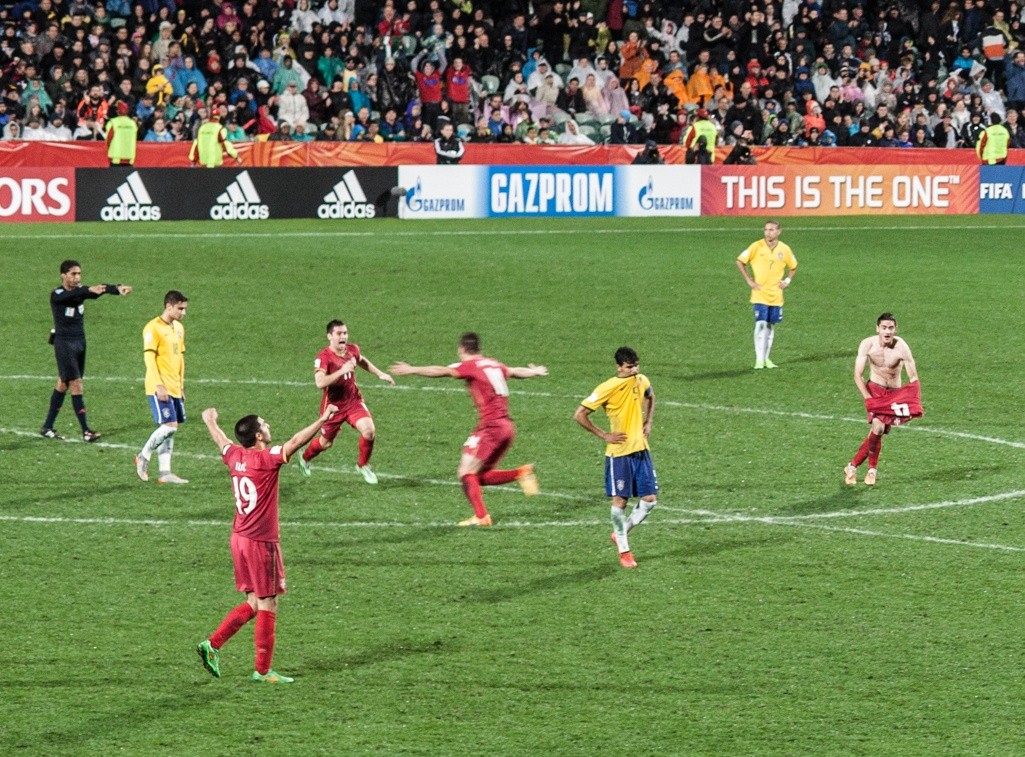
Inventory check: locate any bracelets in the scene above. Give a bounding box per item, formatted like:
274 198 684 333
784 278 791 284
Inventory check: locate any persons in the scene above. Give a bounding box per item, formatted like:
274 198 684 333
135 290 188 484
843 312 923 486
388 331 549 526
196 404 339 682
38 260 132 441
573 346 659 567
736 222 796 369
299 319 395 484
0 0 1025 166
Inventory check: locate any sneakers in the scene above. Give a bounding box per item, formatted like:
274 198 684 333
84 430 102 441
843 462 857 484
865 467 878 486
297 454 312 478
610 533 617 546
252 669 294 684
158 472 188 484
196 638 221 679
753 360 764 369
356 462 378 485
458 514 492 526
516 464 537 497
617 548 637 569
40 426 66 441
135 453 151 482
764 359 780 370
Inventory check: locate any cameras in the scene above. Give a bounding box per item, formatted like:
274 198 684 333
644 149 658 160
739 147 750 158
698 142 706 153
